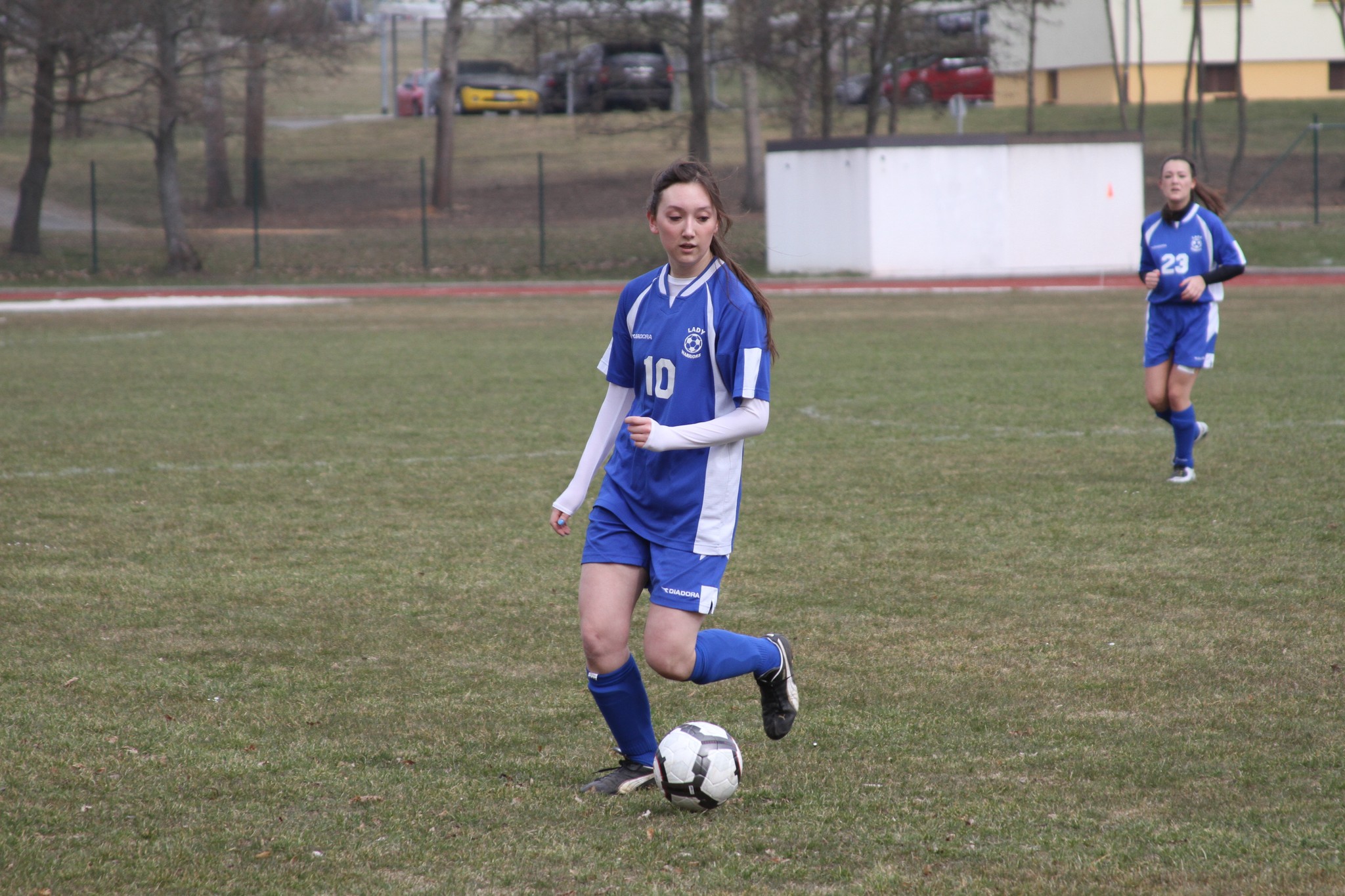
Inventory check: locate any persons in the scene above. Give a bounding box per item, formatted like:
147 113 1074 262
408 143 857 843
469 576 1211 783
1139 156 1250 482
547 155 802 793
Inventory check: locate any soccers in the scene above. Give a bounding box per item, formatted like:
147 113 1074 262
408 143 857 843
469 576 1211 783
651 721 744 813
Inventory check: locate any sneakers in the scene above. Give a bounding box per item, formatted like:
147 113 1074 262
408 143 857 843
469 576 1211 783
1167 465 1196 482
754 633 799 739
576 759 656 797
1193 421 1208 444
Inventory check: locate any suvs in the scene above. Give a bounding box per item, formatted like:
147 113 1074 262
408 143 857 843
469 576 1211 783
531 52 585 115
572 43 675 110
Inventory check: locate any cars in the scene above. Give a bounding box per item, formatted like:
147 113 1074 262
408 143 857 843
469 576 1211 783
393 66 472 131
424 58 537 116
870 45 995 100
